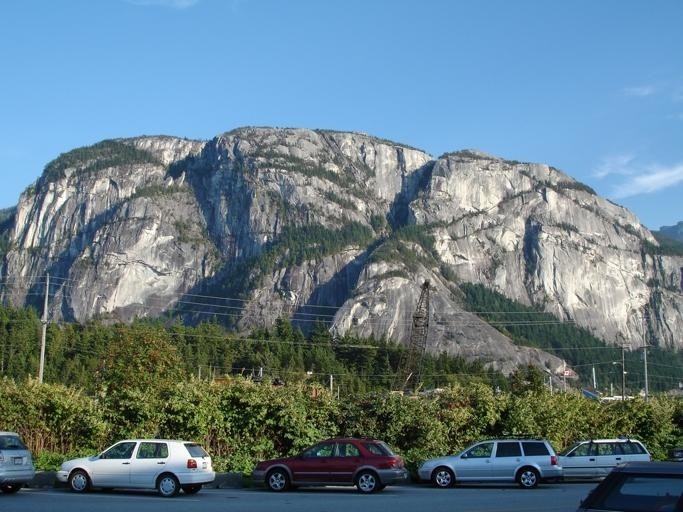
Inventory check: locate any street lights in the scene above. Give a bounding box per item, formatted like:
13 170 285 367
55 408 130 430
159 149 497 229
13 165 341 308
612 359 627 400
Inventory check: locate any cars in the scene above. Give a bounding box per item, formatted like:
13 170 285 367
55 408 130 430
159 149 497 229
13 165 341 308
256 437 408 495
578 461 683 512
418 438 564 490
54 436 216 495
559 437 651 481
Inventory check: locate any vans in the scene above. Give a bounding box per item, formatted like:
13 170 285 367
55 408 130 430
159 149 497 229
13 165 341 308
0 431 35 497
